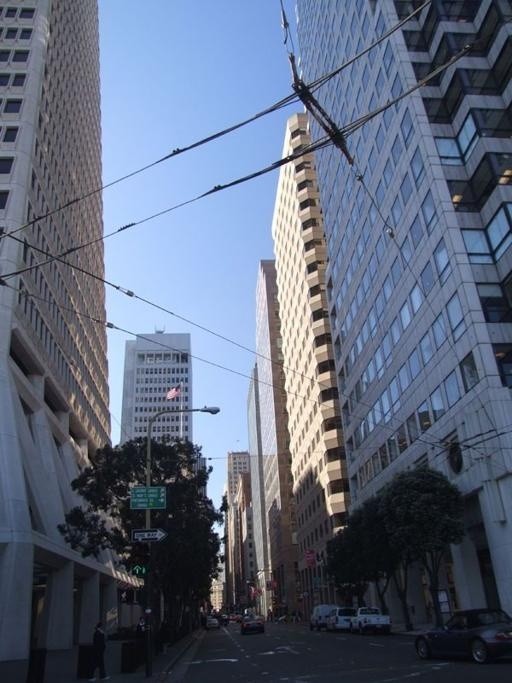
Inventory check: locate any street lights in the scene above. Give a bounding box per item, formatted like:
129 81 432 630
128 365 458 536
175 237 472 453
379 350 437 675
143 405 226 678
164 380 192 439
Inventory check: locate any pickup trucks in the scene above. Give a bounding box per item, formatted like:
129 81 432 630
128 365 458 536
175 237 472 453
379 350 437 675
349 607 393 635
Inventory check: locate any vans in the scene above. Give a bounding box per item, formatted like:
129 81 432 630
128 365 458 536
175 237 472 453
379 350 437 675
309 604 357 632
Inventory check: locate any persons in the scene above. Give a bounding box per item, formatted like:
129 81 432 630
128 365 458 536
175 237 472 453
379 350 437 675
86 622 112 682
136 616 145 631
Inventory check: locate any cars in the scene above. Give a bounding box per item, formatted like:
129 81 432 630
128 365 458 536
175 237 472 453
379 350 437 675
206 613 265 634
415 608 512 664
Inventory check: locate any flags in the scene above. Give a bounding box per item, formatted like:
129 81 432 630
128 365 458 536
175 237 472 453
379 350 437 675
166 382 182 402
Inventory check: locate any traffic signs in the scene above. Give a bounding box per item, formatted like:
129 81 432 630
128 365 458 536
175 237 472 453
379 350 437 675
131 528 169 544
130 486 166 510
